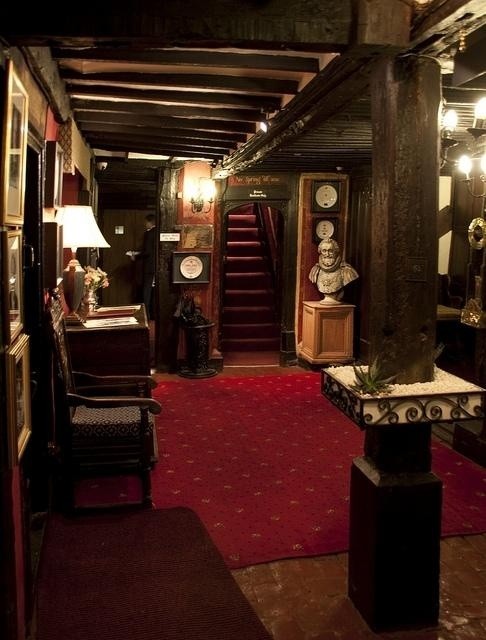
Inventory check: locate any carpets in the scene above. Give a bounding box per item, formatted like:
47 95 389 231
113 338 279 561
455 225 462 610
70 359 485 572
25 497 278 639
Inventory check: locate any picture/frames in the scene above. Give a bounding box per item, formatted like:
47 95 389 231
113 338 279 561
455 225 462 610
9 327 36 465
1 58 30 230
46 136 65 211
172 251 213 287
310 215 339 247
310 175 342 215
78 187 95 209
3 225 26 351
43 218 65 291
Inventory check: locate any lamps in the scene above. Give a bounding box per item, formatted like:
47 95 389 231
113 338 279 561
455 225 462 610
185 176 217 216
455 150 485 204
259 118 273 134
467 96 486 142
55 202 111 326
441 107 455 170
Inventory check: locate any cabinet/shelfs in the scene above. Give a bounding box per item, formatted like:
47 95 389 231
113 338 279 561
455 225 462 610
297 298 357 370
179 321 218 379
65 299 155 401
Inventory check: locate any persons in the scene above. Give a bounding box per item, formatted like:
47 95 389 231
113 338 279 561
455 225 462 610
125 213 158 319
309 235 360 305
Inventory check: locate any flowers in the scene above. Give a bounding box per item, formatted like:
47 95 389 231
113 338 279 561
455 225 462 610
81 259 109 290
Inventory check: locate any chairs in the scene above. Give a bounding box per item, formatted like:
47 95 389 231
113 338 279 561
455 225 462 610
46 301 162 512
436 272 465 358
